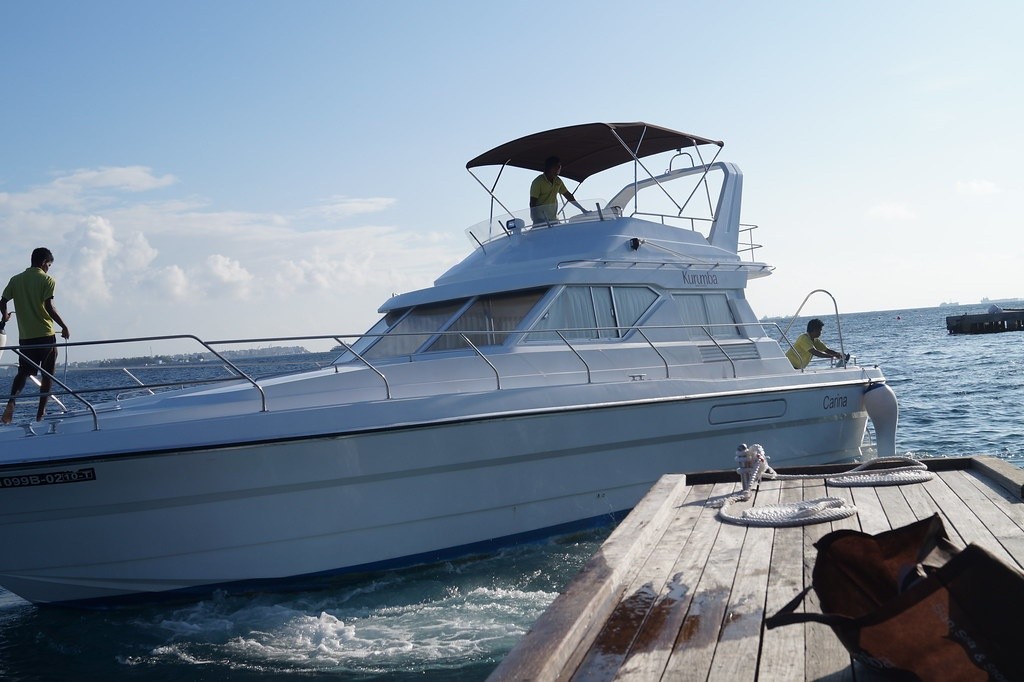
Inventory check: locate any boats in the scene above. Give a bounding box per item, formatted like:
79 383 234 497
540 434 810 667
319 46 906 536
0 122 899 617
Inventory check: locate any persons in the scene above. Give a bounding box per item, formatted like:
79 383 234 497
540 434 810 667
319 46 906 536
0 247 69 424
529 157 593 228
785 319 847 370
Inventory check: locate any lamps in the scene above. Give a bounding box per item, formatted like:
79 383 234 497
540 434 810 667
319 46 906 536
630 237 640 250
506 218 525 234
